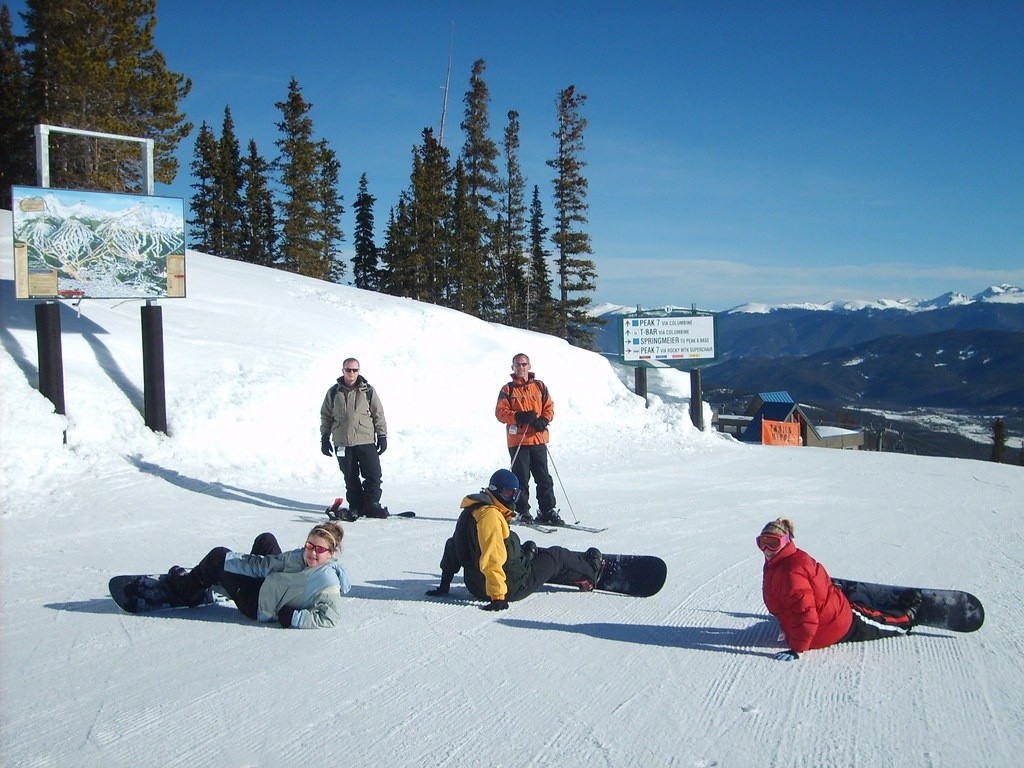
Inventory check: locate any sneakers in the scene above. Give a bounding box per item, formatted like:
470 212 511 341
366 504 389 518
517 510 533 522
349 503 364 519
575 547 602 583
521 540 536 564
538 509 561 523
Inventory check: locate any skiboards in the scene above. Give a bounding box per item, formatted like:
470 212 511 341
510 520 610 534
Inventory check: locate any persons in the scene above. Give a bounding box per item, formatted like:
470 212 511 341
169 522 351 628
757 518 921 661
495 353 562 523
425 469 602 612
320 358 389 519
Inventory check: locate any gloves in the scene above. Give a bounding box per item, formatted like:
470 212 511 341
772 650 799 661
321 439 333 457
376 435 387 455
533 416 549 432
483 599 509 611
515 410 536 424
206 550 226 573
278 605 295 627
425 589 443 597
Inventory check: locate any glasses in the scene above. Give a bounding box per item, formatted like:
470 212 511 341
514 362 528 367
756 533 781 551
500 487 521 503
344 367 359 373
305 541 331 553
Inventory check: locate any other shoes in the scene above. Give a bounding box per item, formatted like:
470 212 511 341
168 566 192 606
902 590 922 628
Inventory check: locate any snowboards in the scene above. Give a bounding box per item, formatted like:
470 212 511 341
829 576 985 634
521 544 668 598
387 511 416 517
107 572 232 614
